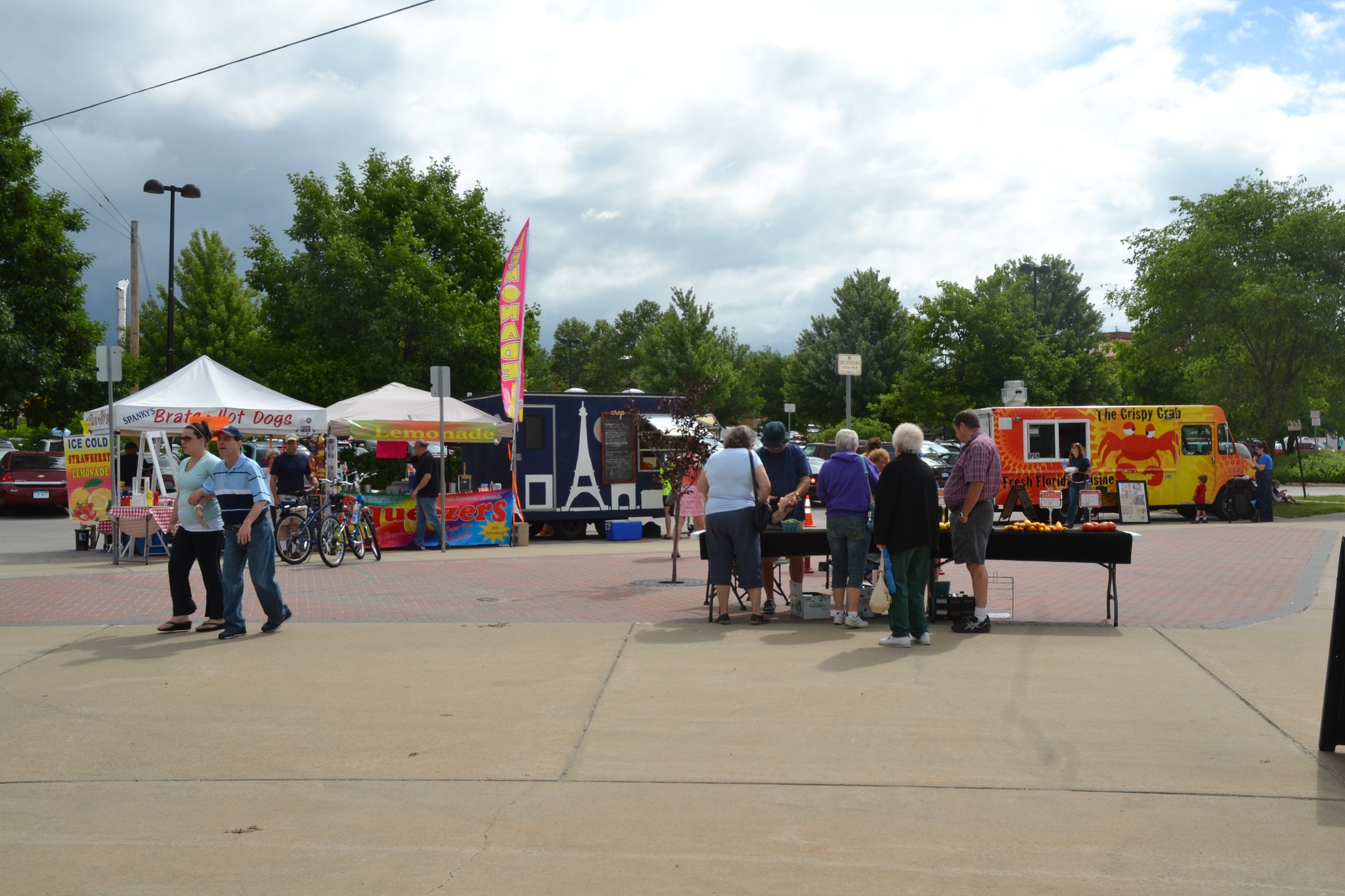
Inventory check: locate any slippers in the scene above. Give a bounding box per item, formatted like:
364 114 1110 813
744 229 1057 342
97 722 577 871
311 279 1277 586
196 621 226 631
662 534 671 539
671 553 681 558
158 621 192 631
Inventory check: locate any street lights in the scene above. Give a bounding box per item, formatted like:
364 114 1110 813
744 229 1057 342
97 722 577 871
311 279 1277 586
560 335 580 390
144 175 203 456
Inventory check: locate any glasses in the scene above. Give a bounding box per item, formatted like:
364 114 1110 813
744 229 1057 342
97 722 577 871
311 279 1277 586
413 446 420 449
1256 448 1261 452
180 436 199 442
877 460 887 465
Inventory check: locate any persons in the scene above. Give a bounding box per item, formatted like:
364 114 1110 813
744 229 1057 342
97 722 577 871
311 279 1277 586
860 437 890 463
1271 478 1303 505
1191 475 1209 523
1249 440 1274 522
659 441 711 559
1063 442 1094 529
193 425 294 641
754 421 813 615
817 428 870 629
874 423 940 648
943 409 1001 635
270 433 320 561
263 446 288 556
407 439 451 550
114 442 153 490
696 424 772 625
639 450 661 482
156 418 226 633
865 447 890 581
918 449 939 613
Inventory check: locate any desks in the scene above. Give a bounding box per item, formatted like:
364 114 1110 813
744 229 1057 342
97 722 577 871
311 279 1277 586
107 505 180 567
699 525 1132 627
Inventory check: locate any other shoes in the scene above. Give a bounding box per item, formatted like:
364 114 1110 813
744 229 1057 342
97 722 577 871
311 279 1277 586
749 614 771 625
1295 501 1302 504
1064 523 1073 529
715 613 730 624
290 552 302 559
281 550 289 561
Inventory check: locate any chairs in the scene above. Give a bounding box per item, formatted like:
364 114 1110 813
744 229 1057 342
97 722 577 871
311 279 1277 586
703 556 790 611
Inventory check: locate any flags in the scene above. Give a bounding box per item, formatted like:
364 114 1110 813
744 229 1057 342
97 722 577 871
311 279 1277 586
500 220 530 423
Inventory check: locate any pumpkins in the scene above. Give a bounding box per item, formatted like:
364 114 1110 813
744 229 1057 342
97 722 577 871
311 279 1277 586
1004 520 1067 532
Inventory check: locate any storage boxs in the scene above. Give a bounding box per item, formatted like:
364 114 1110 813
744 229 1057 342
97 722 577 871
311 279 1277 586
781 520 800 532
605 519 642 541
858 585 882 618
789 580 831 620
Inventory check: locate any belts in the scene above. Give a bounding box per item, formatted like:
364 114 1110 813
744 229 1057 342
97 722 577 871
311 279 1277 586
949 498 991 513
222 513 267 533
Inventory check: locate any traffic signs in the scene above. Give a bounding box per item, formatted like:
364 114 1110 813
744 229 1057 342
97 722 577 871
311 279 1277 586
1287 418 1302 431
1311 418 1321 426
1310 410 1320 418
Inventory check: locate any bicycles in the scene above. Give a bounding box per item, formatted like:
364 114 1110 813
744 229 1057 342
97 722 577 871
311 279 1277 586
317 469 382 569
272 469 358 565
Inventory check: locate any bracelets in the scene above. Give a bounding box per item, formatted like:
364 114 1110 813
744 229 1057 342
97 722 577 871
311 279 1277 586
309 475 317 484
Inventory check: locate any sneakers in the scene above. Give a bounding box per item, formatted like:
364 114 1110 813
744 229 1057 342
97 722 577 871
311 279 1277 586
953 614 990 626
911 631 931 645
1191 520 1199 523
218 625 247 639
951 615 991 633
878 634 911 648
844 613 869 628
402 542 423 551
762 599 776 613
260 604 293 633
833 609 847 624
1202 519 1208 523
431 544 450 550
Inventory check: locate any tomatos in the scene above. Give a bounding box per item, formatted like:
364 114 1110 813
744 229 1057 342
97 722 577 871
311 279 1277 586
1082 521 1116 532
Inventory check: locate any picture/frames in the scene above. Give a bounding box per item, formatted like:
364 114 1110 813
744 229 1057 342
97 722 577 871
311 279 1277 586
1115 480 1151 524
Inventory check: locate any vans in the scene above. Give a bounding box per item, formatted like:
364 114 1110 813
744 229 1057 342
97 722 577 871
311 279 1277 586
1180 440 1253 468
962 403 1259 522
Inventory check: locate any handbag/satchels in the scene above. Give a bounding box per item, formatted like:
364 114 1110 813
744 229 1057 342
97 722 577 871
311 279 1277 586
751 500 770 532
868 547 892 613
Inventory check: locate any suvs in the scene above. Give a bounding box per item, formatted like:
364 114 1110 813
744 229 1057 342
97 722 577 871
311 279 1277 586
233 442 314 483
33 426 72 452
1293 441 1345 454
126 452 183 494
406 441 451 462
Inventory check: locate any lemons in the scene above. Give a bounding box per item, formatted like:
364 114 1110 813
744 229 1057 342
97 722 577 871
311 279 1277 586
70 478 111 510
404 504 442 532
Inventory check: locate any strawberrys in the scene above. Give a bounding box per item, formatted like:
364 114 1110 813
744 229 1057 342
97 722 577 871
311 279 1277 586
70 502 97 521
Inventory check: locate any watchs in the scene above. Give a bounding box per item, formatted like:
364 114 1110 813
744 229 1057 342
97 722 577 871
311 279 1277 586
792 491 801 499
959 512 967 519
193 504 203 511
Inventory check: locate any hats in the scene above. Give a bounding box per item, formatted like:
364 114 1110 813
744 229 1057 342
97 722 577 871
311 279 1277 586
284 433 299 443
125 442 139 452
759 421 790 448
264 453 279 464
213 425 244 442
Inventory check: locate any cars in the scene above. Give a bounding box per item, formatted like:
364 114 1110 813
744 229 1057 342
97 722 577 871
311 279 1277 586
0 438 19 463
0 450 69 512
699 419 961 510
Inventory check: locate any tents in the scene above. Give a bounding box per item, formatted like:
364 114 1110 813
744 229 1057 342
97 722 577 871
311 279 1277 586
324 381 516 547
83 354 328 561
